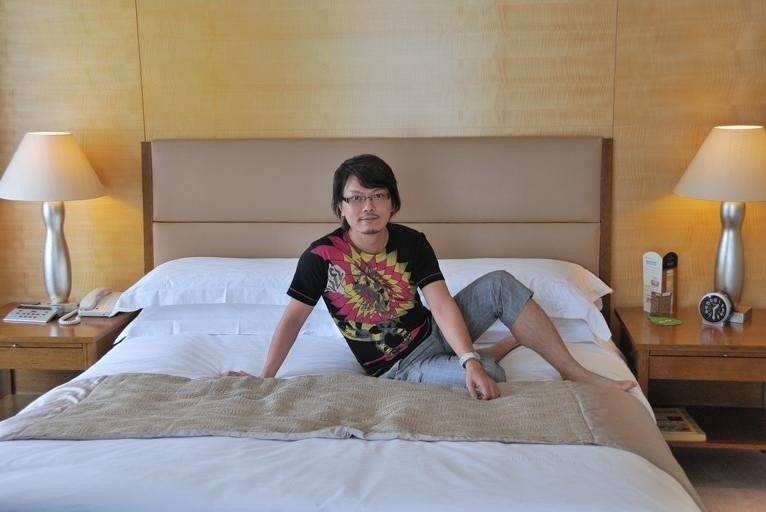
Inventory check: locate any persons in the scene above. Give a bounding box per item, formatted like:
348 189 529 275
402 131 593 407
217 154 637 399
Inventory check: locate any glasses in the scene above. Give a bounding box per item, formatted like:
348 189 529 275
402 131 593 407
337 190 390 205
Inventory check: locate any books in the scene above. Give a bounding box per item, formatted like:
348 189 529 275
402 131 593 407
653 406 705 443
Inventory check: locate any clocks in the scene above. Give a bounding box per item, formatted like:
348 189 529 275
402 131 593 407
699 293 732 328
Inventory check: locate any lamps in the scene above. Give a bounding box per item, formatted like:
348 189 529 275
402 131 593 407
1 133 109 314
673 127 766 325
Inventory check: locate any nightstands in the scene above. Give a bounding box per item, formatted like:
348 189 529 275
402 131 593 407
0 303 141 397
617 307 765 452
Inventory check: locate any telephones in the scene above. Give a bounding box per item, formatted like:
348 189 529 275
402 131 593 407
59 286 124 325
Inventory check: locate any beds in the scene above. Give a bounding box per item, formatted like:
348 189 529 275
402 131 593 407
0 134 709 510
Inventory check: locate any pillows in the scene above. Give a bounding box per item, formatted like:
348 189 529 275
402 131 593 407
110 257 330 314
418 258 614 343
475 316 595 349
111 305 346 345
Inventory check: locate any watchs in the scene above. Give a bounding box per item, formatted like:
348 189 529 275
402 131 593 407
457 350 481 368
